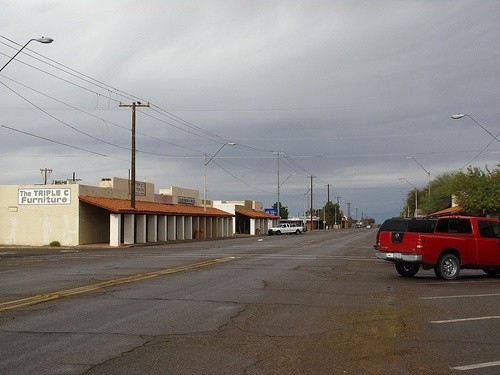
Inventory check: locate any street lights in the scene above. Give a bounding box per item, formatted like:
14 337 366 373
304 185 316 226
276 171 296 218
400 177 418 218
203 142 237 212
405 156 431 202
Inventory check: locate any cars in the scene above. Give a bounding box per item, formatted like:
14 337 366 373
352 224 361 228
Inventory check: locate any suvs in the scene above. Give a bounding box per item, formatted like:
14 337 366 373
373 217 440 252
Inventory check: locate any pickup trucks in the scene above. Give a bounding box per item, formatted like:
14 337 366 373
377 215 500 281
267 224 304 236
